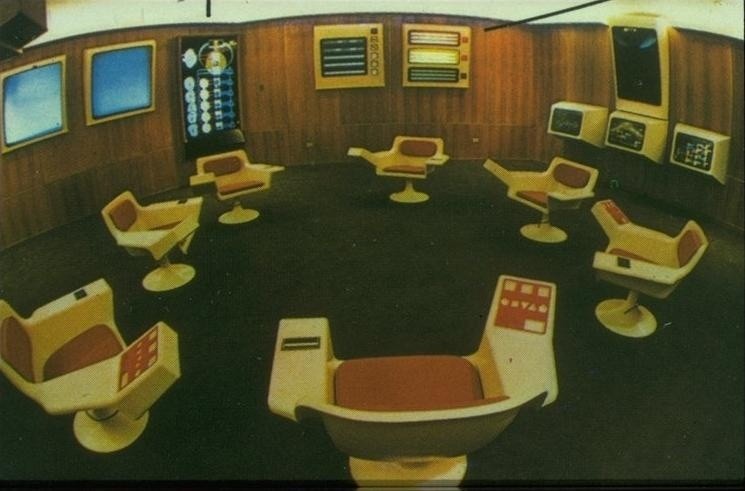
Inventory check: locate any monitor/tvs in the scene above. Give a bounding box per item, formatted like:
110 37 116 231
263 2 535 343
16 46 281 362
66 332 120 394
606 14 671 121
82 39 158 127
0 54 70 155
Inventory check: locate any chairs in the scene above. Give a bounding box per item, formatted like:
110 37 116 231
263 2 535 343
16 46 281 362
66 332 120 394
264 274 563 486
588 199 708 340
482 156 600 244
2 279 184 455
341 135 450 205
191 149 285 224
100 193 203 295
